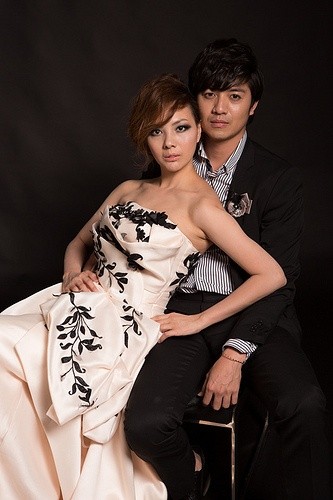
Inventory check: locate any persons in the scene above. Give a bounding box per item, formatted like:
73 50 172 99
124 37 332 499
1 75 287 500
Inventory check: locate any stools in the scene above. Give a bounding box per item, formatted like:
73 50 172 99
184 379 269 499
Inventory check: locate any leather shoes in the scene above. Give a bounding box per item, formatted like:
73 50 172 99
179 452 212 500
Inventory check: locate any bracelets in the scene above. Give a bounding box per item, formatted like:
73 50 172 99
221 351 243 365
